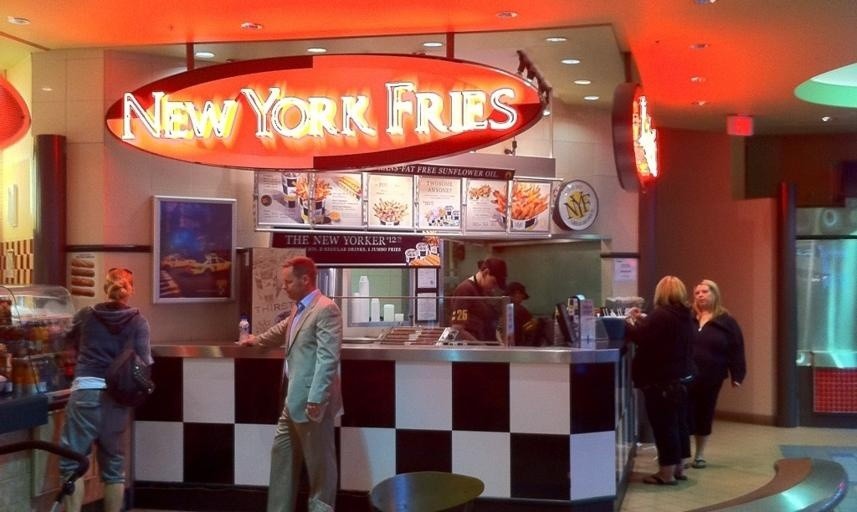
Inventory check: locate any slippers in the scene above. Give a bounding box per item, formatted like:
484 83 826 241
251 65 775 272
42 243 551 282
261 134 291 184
644 457 706 484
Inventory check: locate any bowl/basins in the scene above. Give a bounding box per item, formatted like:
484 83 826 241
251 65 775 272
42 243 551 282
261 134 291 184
497 212 538 231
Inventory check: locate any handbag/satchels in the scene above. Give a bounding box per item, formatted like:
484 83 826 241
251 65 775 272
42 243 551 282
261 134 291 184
106 349 154 406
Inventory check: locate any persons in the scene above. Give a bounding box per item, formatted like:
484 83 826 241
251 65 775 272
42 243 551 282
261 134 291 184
503 281 539 346
627 276 702 486
682 279 747 470
57 265 155 512
448 259 508 348
242 256 349 512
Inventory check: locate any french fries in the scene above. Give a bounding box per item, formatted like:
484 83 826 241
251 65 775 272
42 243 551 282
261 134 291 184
512 182 548 219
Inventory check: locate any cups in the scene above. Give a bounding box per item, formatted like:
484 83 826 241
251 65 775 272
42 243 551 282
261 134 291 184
379 218 401 226
351 275 403 322
427 204 460 227
405 242 439 265
281 173 333 224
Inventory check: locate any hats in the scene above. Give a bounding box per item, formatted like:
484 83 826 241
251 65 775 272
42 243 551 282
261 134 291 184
486 258 508 289
508 281 531 300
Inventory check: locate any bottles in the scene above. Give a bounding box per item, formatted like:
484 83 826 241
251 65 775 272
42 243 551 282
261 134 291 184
0 315 76 395
238 313 249 339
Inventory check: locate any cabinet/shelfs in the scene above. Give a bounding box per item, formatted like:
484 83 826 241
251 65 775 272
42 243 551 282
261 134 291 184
0 282 82 398
